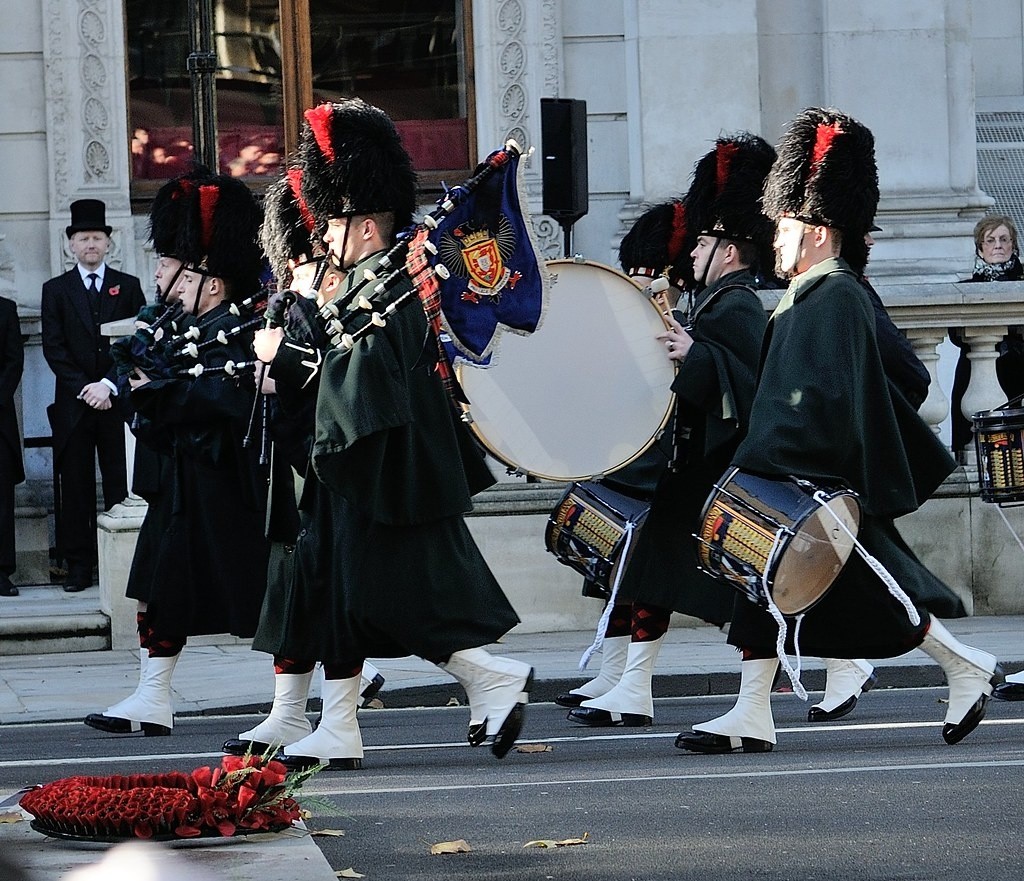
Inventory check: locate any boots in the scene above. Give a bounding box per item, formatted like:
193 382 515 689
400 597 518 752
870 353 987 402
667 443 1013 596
992 670 1024 701
83 647 183 736
262 671 363 769
315 659 385 726
222 666 316 755
555 635 633 707
436 662 492 747
444 646 534 759
808 657 877 720
566 632 666 727
917 612 1003 745
673 657 781 752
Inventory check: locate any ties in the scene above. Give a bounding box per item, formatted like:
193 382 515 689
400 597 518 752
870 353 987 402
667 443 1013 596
87 274 100 310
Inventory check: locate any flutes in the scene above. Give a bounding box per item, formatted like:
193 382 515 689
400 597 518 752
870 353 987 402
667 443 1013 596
150 135 522 468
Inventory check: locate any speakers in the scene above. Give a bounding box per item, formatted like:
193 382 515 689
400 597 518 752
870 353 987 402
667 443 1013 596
540 98 589 229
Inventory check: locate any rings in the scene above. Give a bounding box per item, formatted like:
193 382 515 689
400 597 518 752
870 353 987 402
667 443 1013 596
669 342 673 349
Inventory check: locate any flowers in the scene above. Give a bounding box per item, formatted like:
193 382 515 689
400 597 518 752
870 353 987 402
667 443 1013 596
20 728 357 845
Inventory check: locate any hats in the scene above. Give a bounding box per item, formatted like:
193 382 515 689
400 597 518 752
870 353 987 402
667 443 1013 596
66 199 113 239
620 101 882 292
142 95 417 287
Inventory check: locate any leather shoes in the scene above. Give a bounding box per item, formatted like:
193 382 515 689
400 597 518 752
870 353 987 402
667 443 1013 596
0 578 18 596
64 568 92 591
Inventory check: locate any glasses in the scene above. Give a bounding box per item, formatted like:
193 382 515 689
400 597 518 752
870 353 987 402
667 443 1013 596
981 235 1012 246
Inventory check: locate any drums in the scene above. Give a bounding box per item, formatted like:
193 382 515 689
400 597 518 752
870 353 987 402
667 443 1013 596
689 464 865 620
453 255 680 482
970 408 1024 504
545 481 653 595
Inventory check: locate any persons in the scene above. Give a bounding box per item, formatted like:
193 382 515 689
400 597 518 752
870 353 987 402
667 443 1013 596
0 296 26 596
992 669 1024 702
222 173 488 753
272 98 535 762
567 132 877 727
675 108 1005 755
555 203 881 720
948 214 1024 452
39 199 146 589
84 163 386 752
